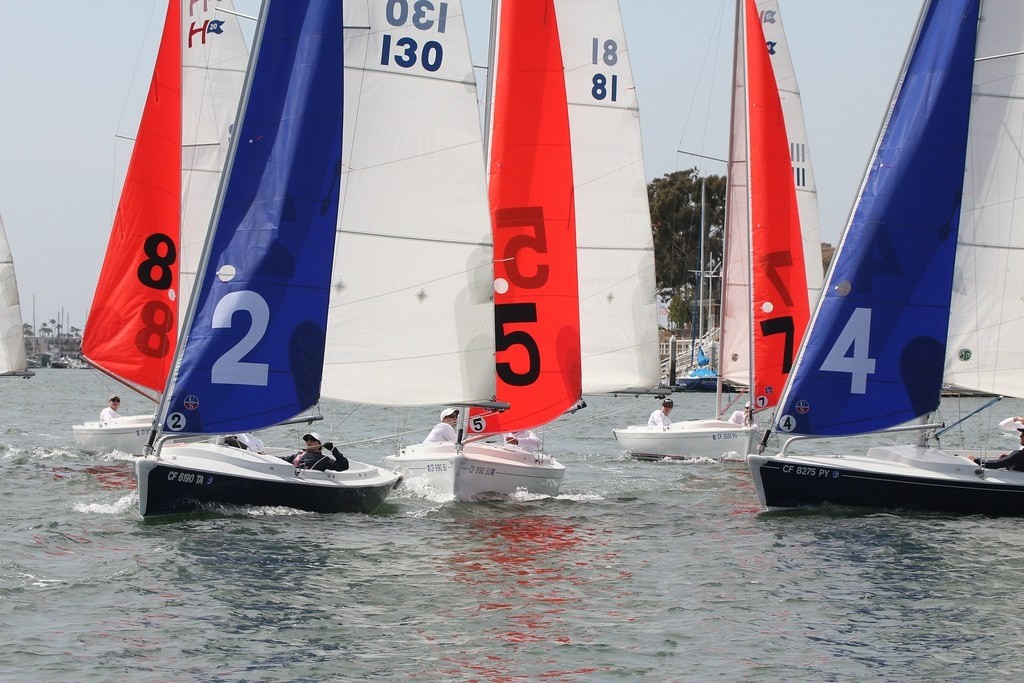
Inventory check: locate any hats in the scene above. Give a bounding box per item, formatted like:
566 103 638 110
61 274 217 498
108 394 120 402
440 408 460 422
302 432 322 444
745 400 754 410
661 398 674 407
1017 427 1024 433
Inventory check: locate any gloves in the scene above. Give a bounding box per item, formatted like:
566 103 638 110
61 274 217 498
323 442 335 452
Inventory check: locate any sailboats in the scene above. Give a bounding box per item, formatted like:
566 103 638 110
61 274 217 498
0 214 95 377
78 0 663 519
613 0 1024 517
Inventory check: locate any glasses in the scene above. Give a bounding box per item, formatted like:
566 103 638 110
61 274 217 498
447 414 458 418
305 437 318 442
745 407 749 409
663 404 672 408
110 398 120 403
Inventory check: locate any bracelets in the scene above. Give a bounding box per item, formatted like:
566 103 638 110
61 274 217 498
516 439 518 445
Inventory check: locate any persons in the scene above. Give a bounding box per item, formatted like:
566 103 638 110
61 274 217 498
99 393 121 421
423 408 459 444
503 430 540 451
275 432 349 471
729 401 755 423
647 399 674 429
968 416 1024 471
